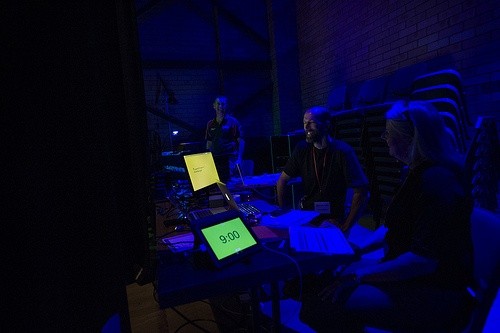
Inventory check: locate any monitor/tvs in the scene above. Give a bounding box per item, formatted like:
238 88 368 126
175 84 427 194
201 216 258 267
183 151 221 197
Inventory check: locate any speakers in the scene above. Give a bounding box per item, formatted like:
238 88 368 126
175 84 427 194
269 130 305 209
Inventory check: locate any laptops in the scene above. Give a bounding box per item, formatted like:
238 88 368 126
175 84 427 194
218 181 261 217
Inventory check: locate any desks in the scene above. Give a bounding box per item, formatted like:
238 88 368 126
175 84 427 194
156 172 357 333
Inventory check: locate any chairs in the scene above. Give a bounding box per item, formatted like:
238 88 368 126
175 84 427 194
269 132 301 210
463 207 499 333
331 69 494 227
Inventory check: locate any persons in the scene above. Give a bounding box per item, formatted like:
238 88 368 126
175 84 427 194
204 96 240 160
302 102 469 333
276 110 370 232
